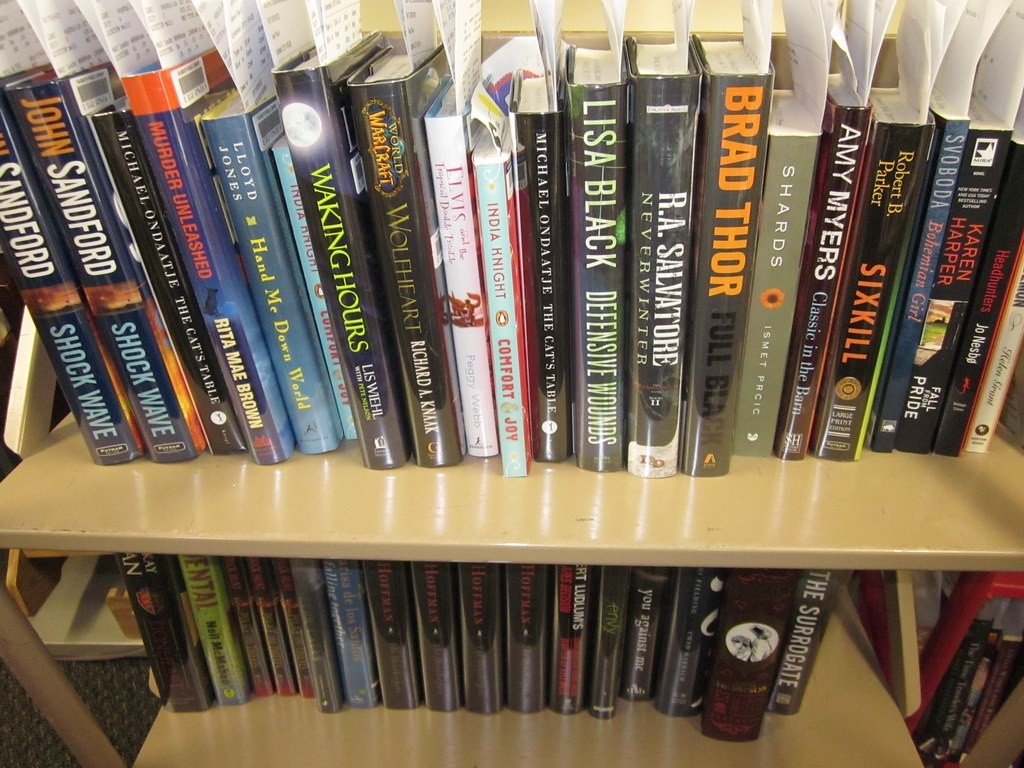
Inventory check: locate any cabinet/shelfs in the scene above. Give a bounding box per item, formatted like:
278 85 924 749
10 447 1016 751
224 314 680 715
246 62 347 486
0 302 1024 768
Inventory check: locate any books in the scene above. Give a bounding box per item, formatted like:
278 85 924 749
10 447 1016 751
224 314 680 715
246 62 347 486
116 553 839 743
0 35 1023 479
848 569 1023 768
